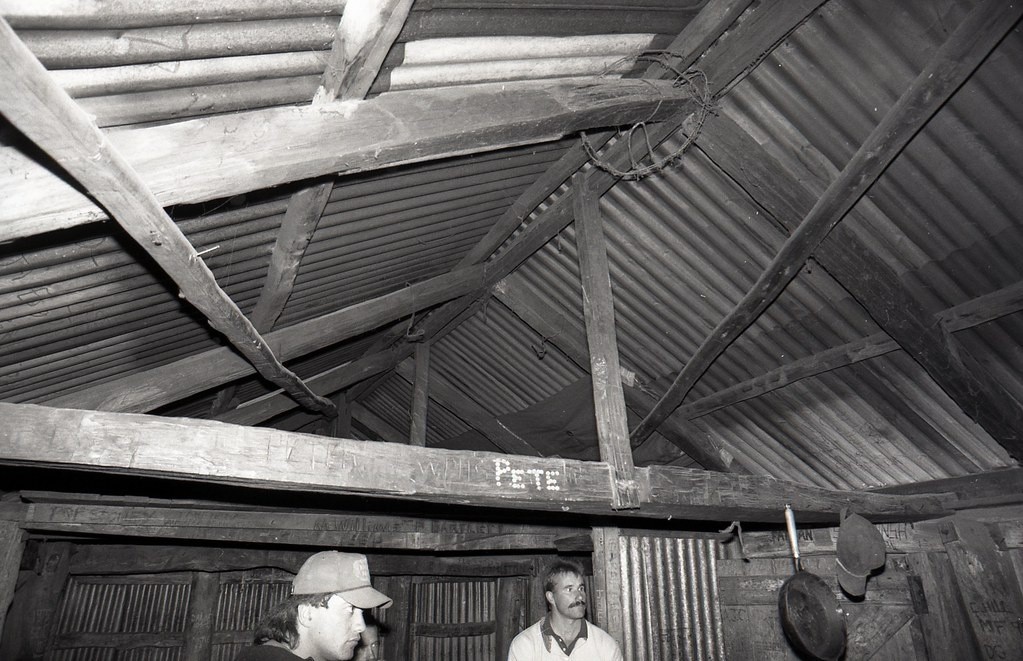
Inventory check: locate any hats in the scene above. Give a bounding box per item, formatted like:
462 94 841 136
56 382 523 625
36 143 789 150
837 506 886 596
291 550 393 609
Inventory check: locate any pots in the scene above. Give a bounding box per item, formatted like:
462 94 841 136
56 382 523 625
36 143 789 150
778 504 848 661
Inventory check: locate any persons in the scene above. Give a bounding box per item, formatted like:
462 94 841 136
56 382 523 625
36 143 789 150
231 550 393 661
507 560 625 661
